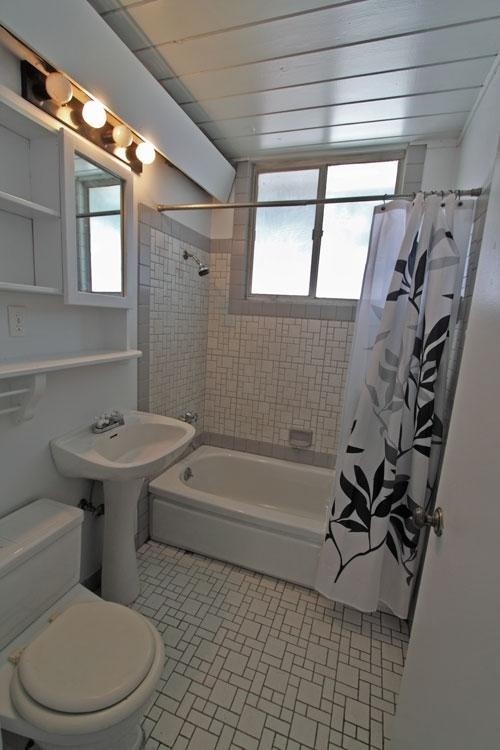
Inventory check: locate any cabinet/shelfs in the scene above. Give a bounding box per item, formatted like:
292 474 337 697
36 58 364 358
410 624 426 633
0 185 60 298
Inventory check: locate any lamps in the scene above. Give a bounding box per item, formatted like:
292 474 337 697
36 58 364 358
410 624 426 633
21 58 159 175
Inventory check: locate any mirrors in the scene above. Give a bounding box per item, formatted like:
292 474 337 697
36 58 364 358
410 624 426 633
58 124 133 309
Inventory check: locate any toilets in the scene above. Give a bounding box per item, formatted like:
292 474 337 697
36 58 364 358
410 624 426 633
0 498 165 749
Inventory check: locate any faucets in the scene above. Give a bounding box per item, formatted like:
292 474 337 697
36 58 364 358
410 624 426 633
179 408 196 422
93 407 122 428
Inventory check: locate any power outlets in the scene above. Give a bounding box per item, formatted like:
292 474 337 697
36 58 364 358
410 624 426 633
8 304 28 338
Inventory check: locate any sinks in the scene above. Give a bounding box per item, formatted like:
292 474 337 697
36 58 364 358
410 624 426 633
49 410 195 482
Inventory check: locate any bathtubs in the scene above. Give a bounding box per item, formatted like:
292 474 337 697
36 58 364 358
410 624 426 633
148 444 336 590
